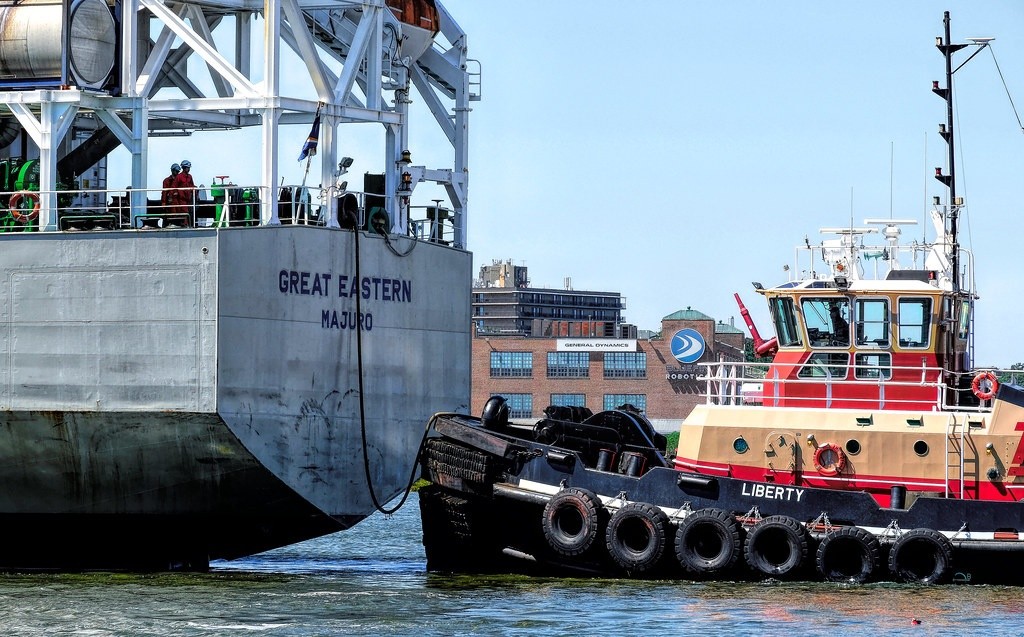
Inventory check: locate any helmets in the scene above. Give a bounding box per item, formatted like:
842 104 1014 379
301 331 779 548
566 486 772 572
181 160 191 167
171 163 181 172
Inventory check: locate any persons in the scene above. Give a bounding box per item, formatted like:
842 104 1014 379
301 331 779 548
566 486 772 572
829 306 848 346
161 160 196 229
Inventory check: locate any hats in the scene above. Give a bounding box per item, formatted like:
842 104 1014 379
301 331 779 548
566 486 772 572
829 307 839 313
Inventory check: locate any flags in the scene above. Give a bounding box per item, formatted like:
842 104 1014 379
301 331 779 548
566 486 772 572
298 107 320 161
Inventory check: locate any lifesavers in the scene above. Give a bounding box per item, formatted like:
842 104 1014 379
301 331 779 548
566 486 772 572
10 193 40 222
816 527 879 582
813 444 846 477
542 487 601 556
744 516 807 575
606 504 667 572
674 508 739 574
889 529 952 586
972 373 998 399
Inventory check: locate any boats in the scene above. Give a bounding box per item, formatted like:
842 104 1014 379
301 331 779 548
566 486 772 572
419 9 1024 587
0 0 481 554
